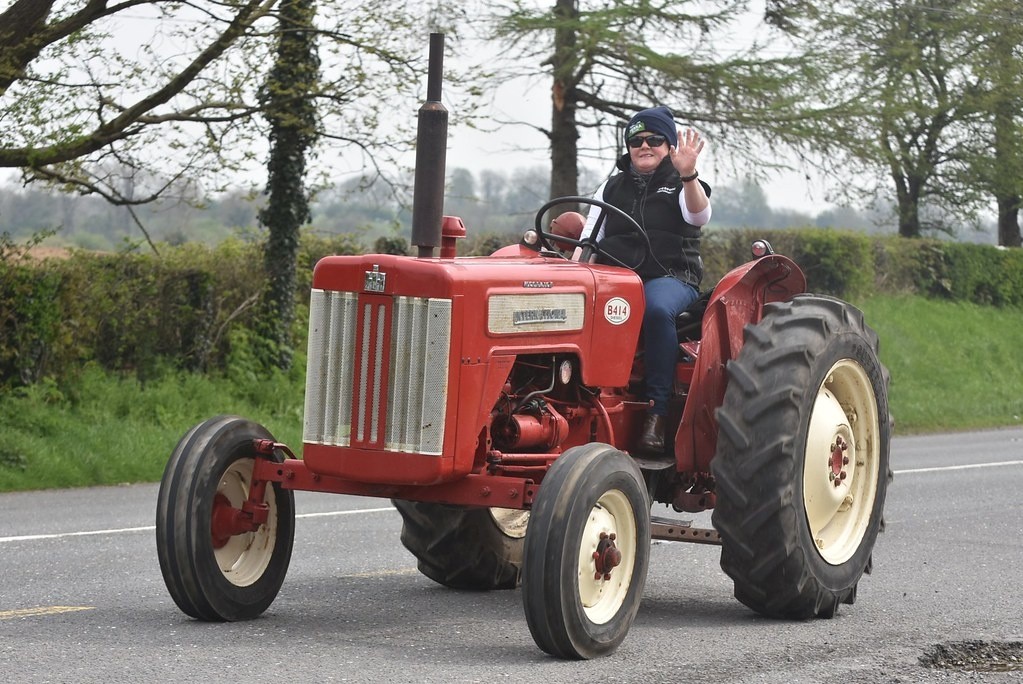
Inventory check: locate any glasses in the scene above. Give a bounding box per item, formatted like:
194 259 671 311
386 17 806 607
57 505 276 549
628 135 667 148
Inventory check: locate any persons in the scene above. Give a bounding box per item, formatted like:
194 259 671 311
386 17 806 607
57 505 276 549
516 106 712 457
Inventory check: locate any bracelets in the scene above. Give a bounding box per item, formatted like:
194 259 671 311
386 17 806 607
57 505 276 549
680 169 698 182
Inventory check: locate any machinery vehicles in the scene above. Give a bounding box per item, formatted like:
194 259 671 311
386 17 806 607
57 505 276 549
153 30 896 662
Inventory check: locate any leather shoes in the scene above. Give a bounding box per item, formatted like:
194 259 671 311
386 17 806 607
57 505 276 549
635 411 666 454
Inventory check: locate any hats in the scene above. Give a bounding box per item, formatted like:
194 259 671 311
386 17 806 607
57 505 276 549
624 106 677 152
